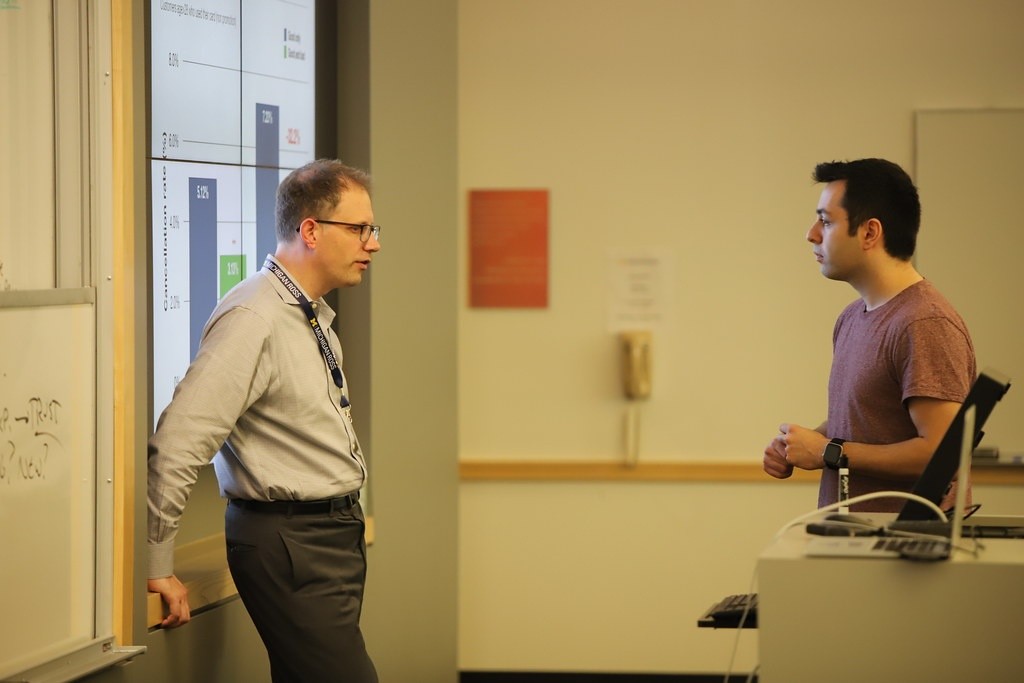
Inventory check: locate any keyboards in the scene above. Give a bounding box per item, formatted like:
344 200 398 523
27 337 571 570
710 593 758 620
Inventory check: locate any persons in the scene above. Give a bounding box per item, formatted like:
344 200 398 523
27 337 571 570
764 158 976 519
147 159 382 683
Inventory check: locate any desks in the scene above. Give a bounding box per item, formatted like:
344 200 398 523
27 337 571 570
753 501 1024 683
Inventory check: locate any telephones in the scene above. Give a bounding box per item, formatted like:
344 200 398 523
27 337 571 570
616 330 653 400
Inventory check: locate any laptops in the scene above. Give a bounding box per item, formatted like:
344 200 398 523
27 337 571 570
802 402 975 559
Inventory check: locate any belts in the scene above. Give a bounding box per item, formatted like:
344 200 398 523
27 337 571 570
231 492 359 513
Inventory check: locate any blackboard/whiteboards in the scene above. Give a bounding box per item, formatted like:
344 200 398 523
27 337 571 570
0 288 115 683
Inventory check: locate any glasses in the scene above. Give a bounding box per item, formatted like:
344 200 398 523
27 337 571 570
296 220 380 242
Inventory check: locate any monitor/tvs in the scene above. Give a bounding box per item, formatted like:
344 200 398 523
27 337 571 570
896 367 1011 523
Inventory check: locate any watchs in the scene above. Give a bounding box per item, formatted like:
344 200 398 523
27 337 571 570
824 438 846 470
